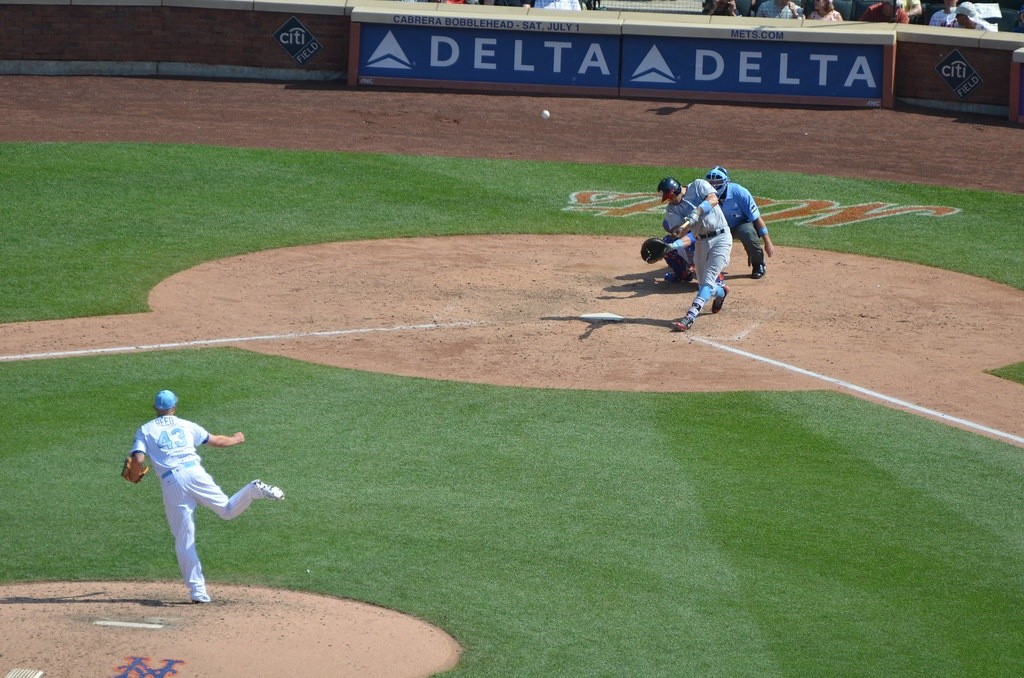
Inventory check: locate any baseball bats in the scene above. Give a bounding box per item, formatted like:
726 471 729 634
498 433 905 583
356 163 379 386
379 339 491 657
673 221 689 237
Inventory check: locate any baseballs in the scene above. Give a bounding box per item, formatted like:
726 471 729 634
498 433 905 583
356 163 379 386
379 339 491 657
541 110 550 119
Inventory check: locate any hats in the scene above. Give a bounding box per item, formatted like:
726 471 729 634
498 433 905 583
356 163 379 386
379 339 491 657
950 1 977 16
155 389 180 410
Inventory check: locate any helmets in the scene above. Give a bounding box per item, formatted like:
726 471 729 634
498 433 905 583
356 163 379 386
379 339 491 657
704 165 729 196
657 176 681 204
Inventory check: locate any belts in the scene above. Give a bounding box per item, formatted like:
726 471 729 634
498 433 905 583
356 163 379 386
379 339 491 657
161 460 199 479
695 229 725 240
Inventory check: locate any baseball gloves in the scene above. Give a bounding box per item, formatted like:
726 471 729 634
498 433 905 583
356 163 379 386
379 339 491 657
640 237 670 264
121 457 150 483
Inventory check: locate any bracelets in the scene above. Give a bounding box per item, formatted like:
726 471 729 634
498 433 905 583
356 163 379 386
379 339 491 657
759 227 768 235
699 200 712 214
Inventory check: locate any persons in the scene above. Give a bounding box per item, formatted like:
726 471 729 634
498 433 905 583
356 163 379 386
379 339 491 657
703 0 1024 36
640 232 725 285
657 177 734 331
704 166 775 280
120 390 285 605
420 0 596 12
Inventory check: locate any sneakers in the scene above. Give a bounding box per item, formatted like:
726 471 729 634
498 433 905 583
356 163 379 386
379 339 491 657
664 272 686 282
712 286 729 314
715 278 725 287
672 315 693 331
254 479 285 500
191 594 210 603
751 263 766 278
